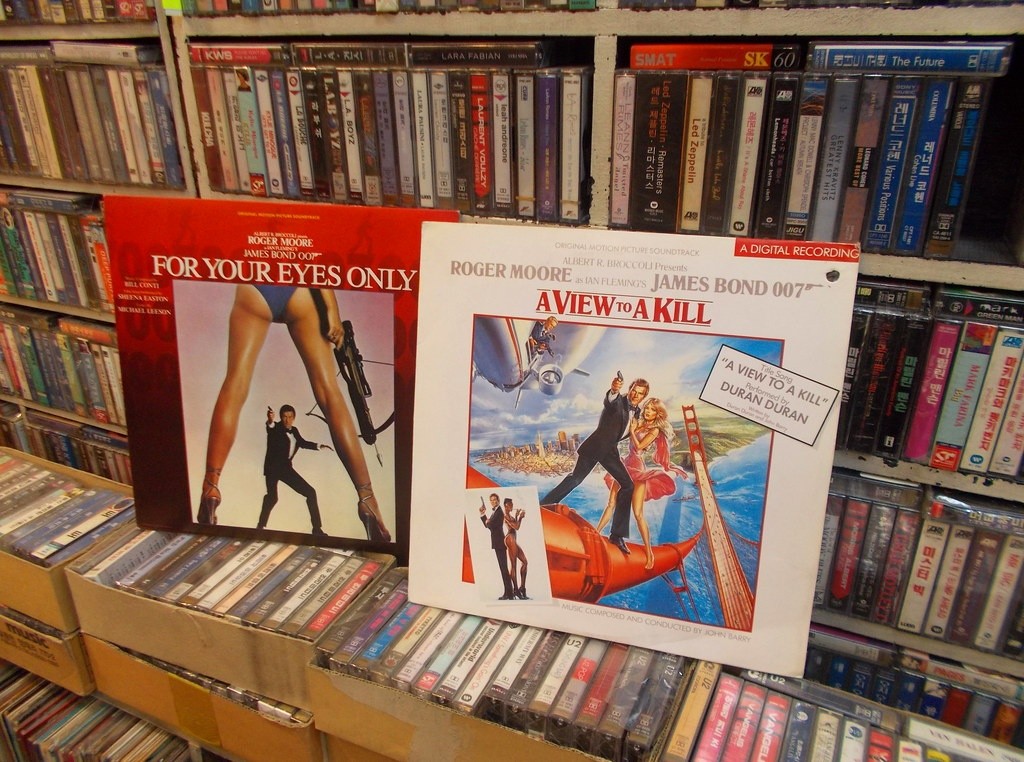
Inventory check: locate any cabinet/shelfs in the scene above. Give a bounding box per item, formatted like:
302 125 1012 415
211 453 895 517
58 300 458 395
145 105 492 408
0 0 1024 762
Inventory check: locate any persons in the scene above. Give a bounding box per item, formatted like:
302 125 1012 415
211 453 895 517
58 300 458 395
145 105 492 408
257 404 334 536
196 283 391 542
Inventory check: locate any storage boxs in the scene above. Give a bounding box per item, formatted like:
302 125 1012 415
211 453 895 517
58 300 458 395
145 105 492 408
0 444 607 762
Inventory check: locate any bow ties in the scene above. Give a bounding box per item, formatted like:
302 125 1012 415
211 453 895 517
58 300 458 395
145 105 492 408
492 507 495 511
628 405 638 412
285 427 293 434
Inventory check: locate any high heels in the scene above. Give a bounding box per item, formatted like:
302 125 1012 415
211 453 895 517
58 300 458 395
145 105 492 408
514 587 531 599
197 468 222 526
356 484 389 542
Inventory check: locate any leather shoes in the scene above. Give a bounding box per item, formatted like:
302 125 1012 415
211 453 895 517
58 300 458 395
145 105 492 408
499 593 514 600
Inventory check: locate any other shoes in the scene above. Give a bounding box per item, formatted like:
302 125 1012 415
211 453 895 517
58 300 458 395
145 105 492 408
312 529 328 536
